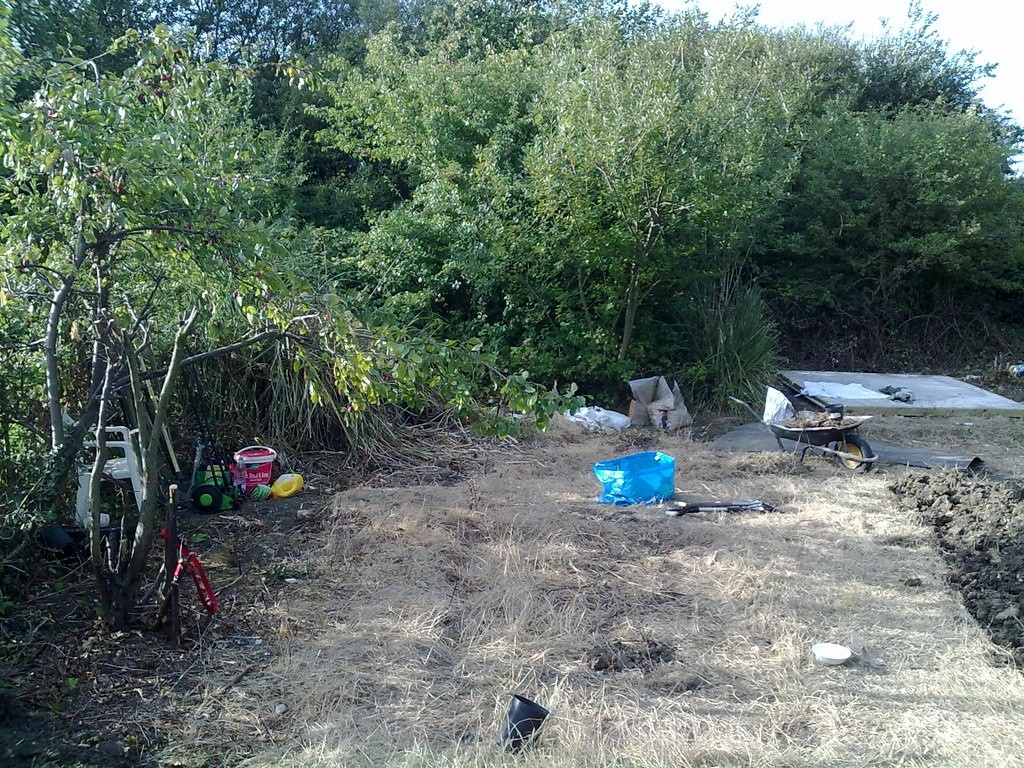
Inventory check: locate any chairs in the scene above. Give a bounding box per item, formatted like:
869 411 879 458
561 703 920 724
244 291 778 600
76 426 142 524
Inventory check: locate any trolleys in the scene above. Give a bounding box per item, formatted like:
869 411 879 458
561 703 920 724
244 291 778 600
729 386 878 474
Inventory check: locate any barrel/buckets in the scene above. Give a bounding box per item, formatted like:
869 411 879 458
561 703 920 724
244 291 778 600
825 403 844 424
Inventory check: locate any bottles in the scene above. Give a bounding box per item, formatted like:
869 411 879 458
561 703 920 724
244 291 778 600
234 457 247 495
